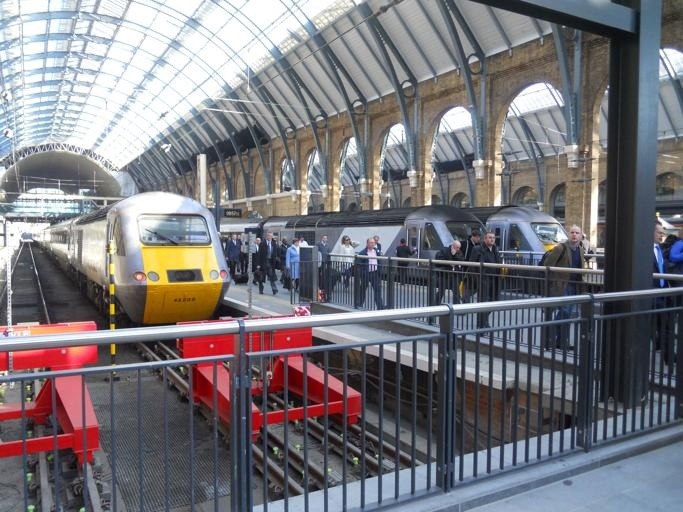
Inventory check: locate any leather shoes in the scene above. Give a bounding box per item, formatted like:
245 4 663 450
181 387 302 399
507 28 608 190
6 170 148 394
260 290 278 295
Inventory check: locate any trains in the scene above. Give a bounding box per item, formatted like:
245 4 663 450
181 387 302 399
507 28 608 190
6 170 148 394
41 192 230 326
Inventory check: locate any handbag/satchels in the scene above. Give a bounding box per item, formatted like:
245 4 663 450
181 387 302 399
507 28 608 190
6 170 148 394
283 276 294 288
538 244 566 265
253 269 263 282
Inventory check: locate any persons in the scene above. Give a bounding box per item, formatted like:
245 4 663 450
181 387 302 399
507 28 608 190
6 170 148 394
542 225 592 351
220 230 388 310
395 238 418 286
652 222 683 365
436 230 503 337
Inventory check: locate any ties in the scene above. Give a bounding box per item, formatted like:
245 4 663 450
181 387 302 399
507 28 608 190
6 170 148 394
655 245 665 287
268 242 270 258
